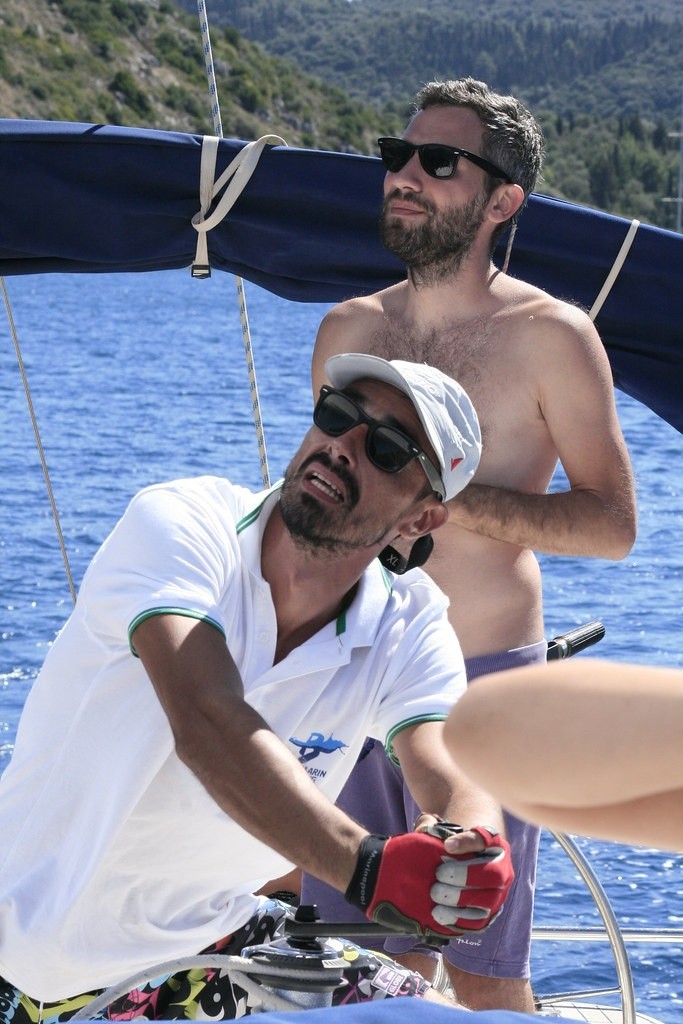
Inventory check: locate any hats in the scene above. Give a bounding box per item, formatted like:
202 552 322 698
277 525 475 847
324 352 482 502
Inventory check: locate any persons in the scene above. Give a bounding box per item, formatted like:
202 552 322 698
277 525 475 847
300 75 640 1014
441 658 683 854
0 353 515 1024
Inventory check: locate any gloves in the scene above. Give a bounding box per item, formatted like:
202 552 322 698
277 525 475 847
342 814 514 941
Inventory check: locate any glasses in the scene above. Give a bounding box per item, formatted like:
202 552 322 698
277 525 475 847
375 132 511 191
312 382 446 505
